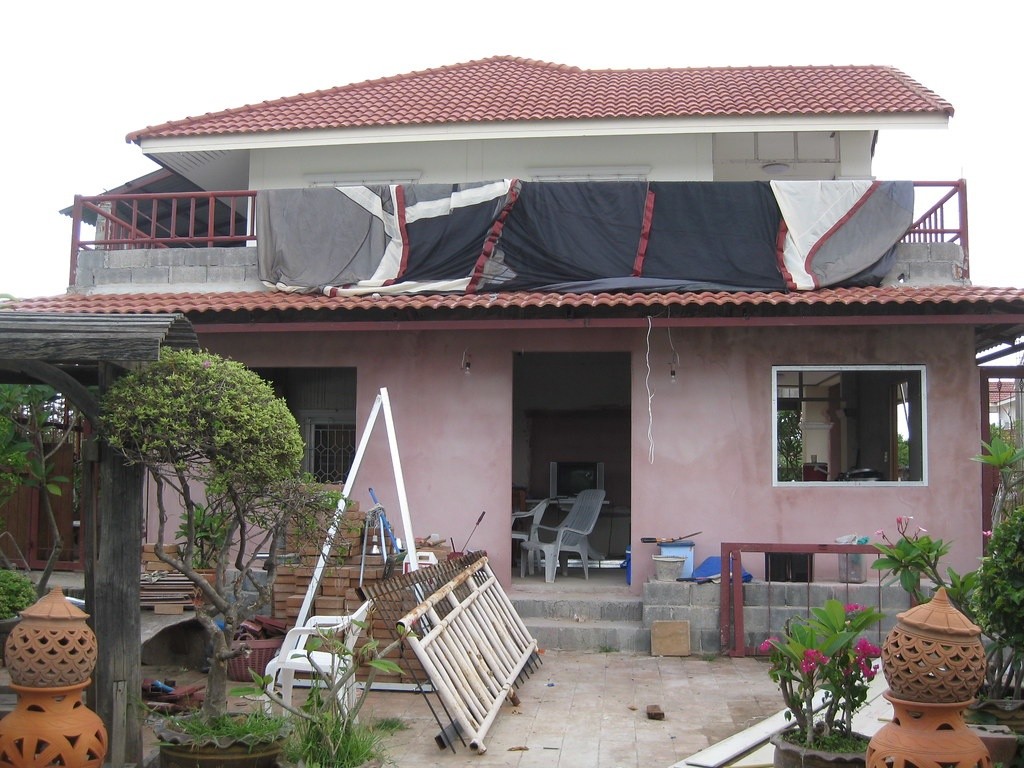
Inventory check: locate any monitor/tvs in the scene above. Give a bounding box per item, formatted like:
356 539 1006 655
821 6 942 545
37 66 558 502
550 461 605 500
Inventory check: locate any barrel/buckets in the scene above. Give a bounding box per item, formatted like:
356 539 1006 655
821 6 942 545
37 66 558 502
620 545 631 585
651 554 687 582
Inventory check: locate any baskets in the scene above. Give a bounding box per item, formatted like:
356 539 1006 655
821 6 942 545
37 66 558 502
226 633 282 682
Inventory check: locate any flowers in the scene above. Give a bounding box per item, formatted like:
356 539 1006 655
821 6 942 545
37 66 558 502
761 597 880 745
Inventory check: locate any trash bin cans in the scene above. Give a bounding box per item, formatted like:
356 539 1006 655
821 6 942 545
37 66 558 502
839 544 867 584
657 540 695 579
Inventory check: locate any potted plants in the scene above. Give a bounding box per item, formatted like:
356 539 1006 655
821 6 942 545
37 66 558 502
0 570 39 667
98 345 365 767
227 600 419 768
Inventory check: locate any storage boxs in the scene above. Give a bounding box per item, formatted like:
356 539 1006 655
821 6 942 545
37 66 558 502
838 553 867 583
766 552 815 582
802 462 828 481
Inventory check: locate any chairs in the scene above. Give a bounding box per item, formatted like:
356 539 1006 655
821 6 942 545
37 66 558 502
263 600 373 724
520 489 606 584
510 497 549 575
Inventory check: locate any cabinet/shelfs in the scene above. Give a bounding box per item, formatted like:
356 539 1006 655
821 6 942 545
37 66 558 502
523 502 630 559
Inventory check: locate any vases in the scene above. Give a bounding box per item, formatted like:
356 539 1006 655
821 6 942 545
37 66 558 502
769 726 872 767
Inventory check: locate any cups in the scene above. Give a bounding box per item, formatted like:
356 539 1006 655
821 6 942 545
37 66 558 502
811 454 817 464
